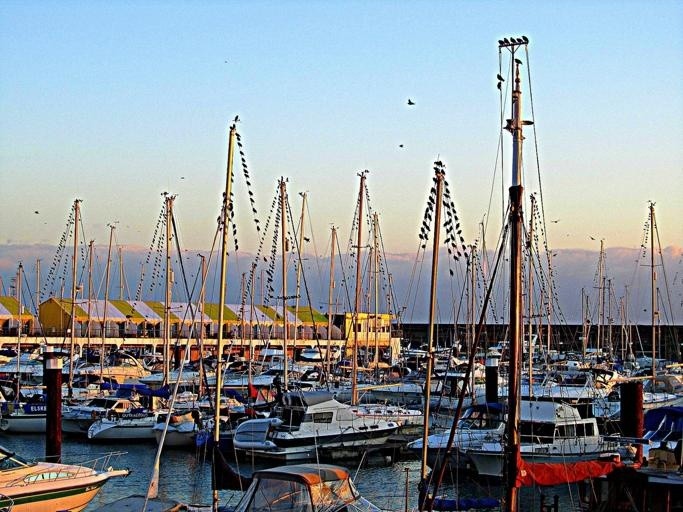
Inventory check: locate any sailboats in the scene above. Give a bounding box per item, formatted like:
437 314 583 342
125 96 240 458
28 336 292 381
0 33 682 511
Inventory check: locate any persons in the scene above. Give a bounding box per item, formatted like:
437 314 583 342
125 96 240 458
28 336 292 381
448 348 468 398
272 372 282 395
392 364 402 379
